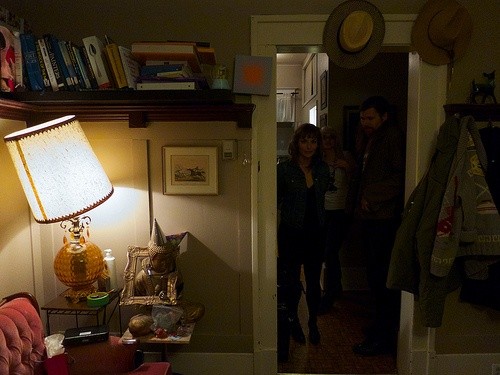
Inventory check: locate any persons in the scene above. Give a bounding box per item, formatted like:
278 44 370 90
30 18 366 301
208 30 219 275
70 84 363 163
278 123 330 344
353 97 404 356
320 125 354 314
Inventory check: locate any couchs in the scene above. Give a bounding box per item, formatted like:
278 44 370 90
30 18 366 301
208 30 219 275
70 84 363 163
0 291 171 375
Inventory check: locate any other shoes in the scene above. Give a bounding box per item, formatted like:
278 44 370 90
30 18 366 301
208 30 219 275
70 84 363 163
308 325 321 344
292 325 306 344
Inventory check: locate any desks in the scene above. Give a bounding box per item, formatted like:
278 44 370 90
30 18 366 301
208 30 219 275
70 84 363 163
40 289 123 339
117 323 197 375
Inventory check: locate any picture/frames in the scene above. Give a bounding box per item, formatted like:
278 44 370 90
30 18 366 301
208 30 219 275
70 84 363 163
120 246 182 305
320 70 327 110
160 144 221 197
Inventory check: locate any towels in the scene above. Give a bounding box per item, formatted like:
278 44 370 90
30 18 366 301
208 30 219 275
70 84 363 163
275 89 294 125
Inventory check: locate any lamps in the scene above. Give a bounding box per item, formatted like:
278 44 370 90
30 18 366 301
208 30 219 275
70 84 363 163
3 114 115 302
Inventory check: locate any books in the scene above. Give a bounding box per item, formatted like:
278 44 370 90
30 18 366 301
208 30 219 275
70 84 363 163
0 22 218 93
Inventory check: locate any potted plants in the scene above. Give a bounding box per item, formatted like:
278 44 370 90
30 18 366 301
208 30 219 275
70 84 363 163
207 61 229 89
